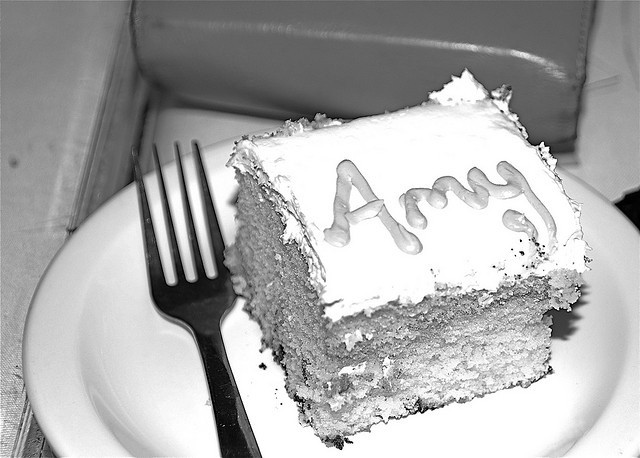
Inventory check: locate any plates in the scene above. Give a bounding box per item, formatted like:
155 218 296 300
23 130 638 458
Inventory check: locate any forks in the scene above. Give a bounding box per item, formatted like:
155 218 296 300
130 137 264 458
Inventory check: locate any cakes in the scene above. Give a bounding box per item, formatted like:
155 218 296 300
222 65 594 451
